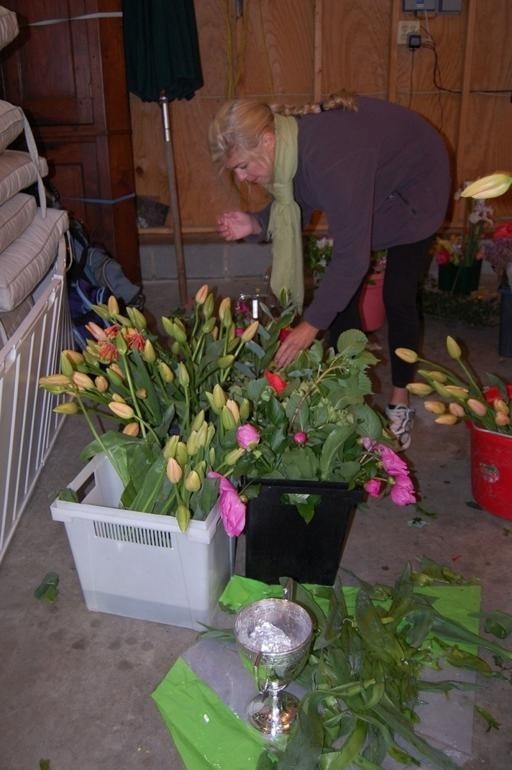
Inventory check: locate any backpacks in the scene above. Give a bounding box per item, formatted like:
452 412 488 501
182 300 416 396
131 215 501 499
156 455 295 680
61 215 146 355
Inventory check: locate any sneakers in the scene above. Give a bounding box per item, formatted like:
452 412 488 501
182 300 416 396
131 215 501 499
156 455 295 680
384 403 417 454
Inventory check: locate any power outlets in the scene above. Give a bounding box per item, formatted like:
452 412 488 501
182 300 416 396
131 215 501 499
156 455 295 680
395 20 420 46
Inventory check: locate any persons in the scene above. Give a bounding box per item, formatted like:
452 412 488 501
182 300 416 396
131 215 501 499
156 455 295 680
208 90 457 454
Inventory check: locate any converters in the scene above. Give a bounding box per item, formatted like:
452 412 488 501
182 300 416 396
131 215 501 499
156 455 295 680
407 32 421 48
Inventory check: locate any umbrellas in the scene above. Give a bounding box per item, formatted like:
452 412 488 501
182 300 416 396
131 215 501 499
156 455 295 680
117 1 203 324
